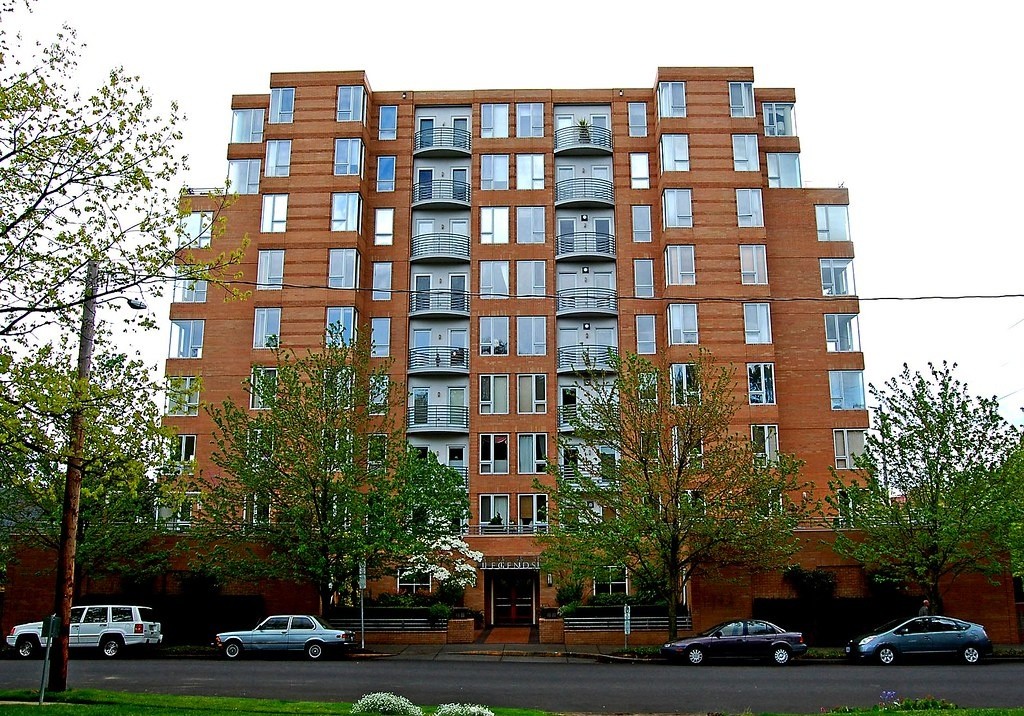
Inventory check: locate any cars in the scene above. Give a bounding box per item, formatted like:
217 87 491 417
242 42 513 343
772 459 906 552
214 615 357 661
845 615 988 665
661 619 808 666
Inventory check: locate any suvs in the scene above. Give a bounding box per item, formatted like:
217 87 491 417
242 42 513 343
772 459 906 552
7 605 163 659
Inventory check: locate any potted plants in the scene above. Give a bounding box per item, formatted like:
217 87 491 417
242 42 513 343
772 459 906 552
577 117 593 144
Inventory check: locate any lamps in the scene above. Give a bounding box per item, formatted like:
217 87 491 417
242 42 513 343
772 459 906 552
582 267 589 273
437 391 440 398
581 168 585 173
441 121 445 126
438 277 442 284
402 92 406 99
584 379 591 386
589 450 592 455
583 223 587 228
438 334 441 340
584 277 587 282
440 223 444 230
471 501 595 589
620 89 624 97
586 333 588 338
436 451 439 456
583 323 590 331
441 171 444 177
803 491 807 498
583 213 586 219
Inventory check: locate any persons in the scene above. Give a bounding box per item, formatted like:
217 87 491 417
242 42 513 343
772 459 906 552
919 599 930 617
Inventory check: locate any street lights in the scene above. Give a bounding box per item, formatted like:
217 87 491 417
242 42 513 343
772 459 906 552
49 293 148 692
